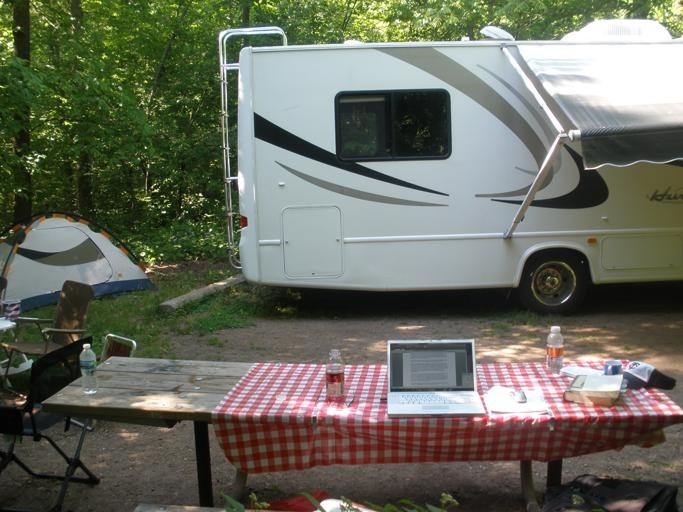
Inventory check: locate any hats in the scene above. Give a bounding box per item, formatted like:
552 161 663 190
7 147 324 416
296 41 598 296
622 361 677 390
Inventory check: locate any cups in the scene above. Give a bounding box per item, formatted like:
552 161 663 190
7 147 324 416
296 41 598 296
604 359 622 375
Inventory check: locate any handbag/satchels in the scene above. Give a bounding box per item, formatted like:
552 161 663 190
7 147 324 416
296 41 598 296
544 474 680 512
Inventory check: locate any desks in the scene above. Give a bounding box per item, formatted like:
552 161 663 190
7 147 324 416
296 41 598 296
42 356 683 511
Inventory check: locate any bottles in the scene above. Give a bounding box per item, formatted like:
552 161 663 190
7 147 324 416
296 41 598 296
325 348 347 403
546 325 565 373
79 343 98 395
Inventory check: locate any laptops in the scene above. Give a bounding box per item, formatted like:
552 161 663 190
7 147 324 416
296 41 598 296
386 338 486 418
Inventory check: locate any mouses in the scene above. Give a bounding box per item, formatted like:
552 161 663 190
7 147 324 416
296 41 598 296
508 385 528 403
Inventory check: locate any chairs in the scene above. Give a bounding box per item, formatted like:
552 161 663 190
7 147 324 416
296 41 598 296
1 279 138 510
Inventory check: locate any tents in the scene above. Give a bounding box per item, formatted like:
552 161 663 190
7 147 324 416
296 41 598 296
0 211 158 316
